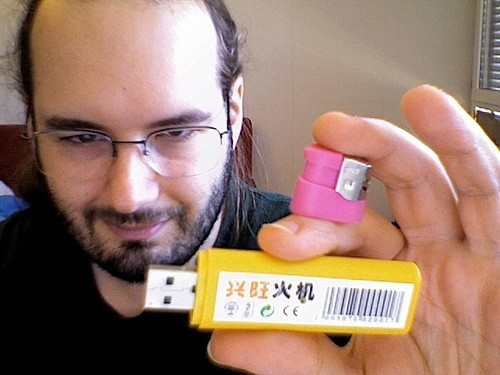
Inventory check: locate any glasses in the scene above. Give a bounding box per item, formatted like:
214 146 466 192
20 88 233 182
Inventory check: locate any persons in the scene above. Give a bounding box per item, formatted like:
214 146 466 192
0 0 500 375
